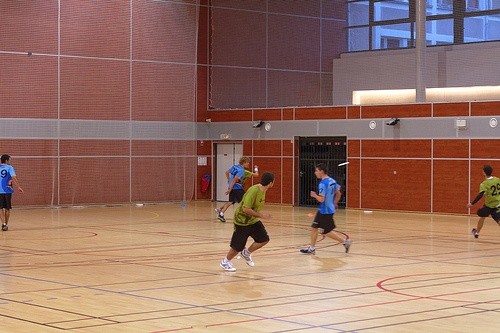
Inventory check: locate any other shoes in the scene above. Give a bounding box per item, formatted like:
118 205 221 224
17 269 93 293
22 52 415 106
1 223 8 231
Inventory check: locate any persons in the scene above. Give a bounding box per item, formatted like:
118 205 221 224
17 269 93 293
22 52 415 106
467 165 500 239
299 163 351 255
215 156 258 222
219 173 276 271
0 154 23 231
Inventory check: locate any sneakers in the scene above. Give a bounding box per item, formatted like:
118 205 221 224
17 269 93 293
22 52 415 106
299 246 316 256
220 258 237 272
240 249 255 267
472 228 479 238
343 239 352 253
215 208 226 222
